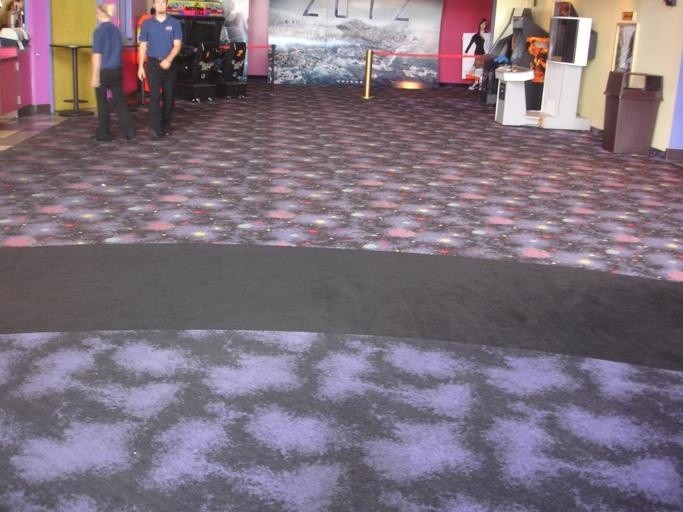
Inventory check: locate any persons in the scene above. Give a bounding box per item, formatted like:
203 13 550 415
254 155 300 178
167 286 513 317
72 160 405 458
91 4 138 145
464 18 489 90
136 0 184 140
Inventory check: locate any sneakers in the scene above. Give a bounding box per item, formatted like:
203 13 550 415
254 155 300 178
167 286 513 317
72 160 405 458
472 83 482 91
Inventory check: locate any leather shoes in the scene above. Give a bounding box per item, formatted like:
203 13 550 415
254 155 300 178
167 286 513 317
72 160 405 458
164 128 171 137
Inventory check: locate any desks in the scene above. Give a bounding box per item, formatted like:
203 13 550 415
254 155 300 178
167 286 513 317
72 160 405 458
49 43 152 118
